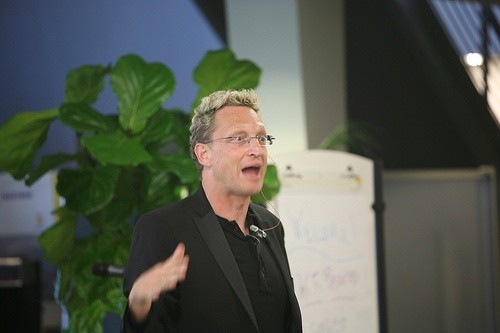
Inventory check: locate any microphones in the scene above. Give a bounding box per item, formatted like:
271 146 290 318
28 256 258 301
250 225 268 238
92 263 125 276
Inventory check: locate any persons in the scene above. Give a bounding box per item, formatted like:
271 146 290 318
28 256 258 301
123 88 304 333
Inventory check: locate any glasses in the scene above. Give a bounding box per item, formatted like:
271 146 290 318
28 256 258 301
204 134 273 146
